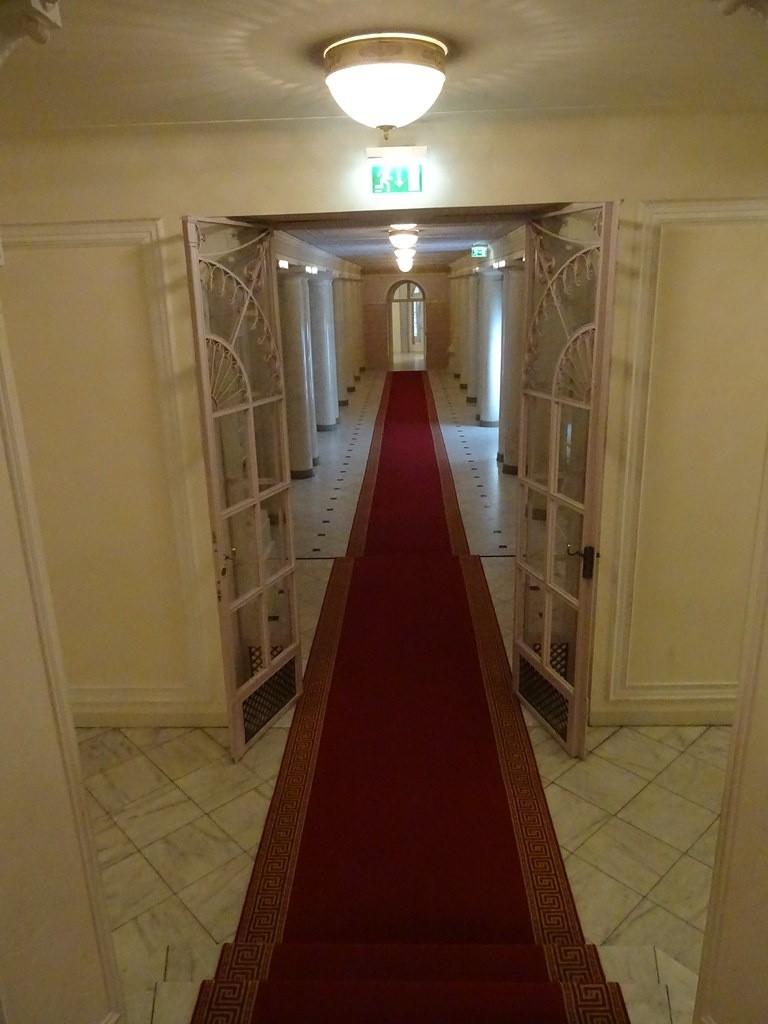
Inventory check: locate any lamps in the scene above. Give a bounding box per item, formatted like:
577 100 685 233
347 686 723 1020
324 31 448 140
398 263 413 273
394 248 417 258
389 232 419 248
389 223 418 230
396 258 414 263
